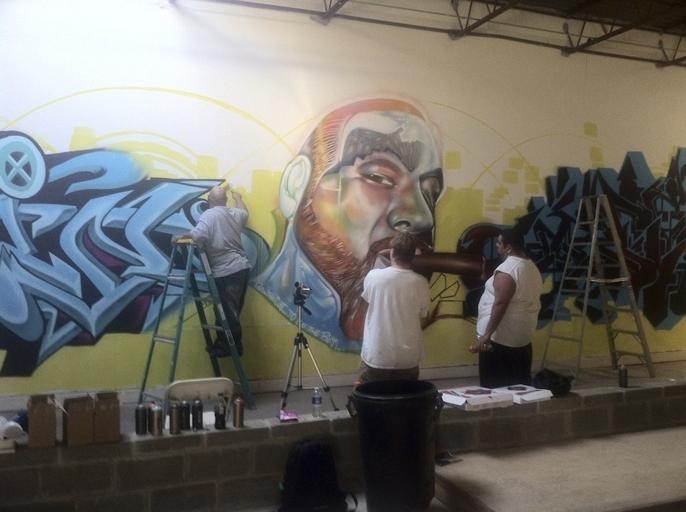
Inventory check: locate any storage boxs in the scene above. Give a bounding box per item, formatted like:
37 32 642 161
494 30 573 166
26 391 121 448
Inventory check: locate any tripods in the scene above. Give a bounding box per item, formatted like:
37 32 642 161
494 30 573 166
280 305 339 412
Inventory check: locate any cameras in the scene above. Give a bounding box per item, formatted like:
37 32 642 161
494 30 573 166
293 282 311 304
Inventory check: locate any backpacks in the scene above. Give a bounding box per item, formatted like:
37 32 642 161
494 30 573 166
278 440 357 512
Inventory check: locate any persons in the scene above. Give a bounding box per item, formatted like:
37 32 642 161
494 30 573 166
355 230 434 386
470 227 542 390
244 97 448 353
169 184 253 359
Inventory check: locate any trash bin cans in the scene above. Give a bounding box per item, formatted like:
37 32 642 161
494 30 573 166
346 379 445 512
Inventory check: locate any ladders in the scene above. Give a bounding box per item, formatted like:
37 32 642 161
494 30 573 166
541 194 655 381
138 238 257 430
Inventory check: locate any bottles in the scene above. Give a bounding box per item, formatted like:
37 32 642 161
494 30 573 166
135 400 162 436
180 400 192 432
192 397 203 429
212 391 227 431
231 395 245 428
311 387 322 418
168 403 181 434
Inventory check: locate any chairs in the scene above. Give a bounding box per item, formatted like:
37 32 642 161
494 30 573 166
164 376 234 428
